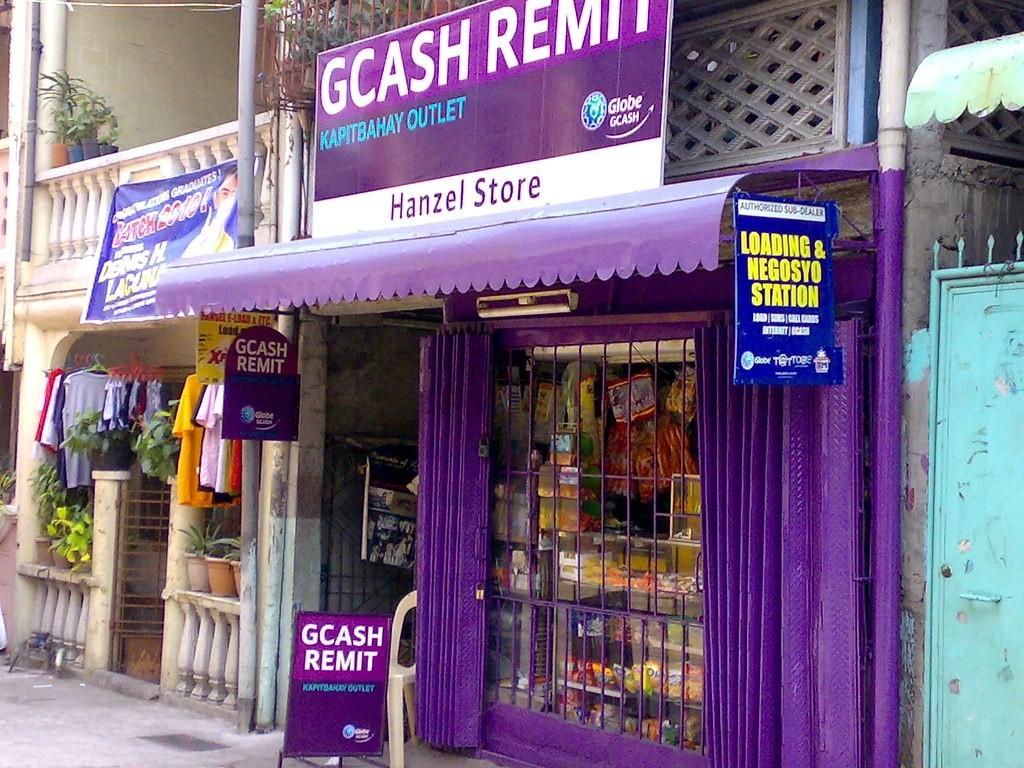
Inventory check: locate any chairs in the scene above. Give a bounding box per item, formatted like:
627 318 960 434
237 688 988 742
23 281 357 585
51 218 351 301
326 589 417 768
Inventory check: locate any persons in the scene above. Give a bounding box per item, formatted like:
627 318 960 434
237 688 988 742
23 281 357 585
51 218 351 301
181 164 239 258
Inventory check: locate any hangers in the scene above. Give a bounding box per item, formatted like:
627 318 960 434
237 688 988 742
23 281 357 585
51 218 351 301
60 348 171 380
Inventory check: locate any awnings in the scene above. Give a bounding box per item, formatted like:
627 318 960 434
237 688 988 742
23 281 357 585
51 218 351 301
903 32 1024 130
154 141 880 318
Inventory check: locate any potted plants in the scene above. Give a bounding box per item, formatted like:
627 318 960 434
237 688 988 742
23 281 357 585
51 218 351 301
27 461 92 574
178 521 241 599
35 70 118 168
58 411 143 470
